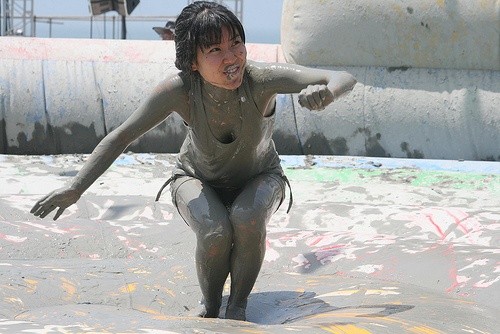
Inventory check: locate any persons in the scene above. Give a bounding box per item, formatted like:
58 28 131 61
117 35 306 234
29 1 358 323
